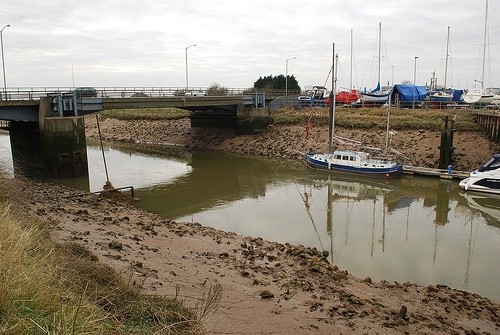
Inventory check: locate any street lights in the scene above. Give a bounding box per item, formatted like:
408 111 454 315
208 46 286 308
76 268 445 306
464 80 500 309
185 44 196 92
0 25 11 100
286 57 296 95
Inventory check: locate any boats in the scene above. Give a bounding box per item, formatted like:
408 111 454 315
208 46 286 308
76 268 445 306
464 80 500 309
458 154 500 195
403 165 471 180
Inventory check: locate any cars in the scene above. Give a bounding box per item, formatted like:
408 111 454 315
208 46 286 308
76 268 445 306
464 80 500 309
185 90 207 97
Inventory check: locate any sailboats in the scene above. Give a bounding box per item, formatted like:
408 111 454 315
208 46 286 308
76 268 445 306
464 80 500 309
307 88 403 179
298 0 500 114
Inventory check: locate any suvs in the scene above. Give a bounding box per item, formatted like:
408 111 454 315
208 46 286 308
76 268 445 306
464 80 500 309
72 86 97 97
